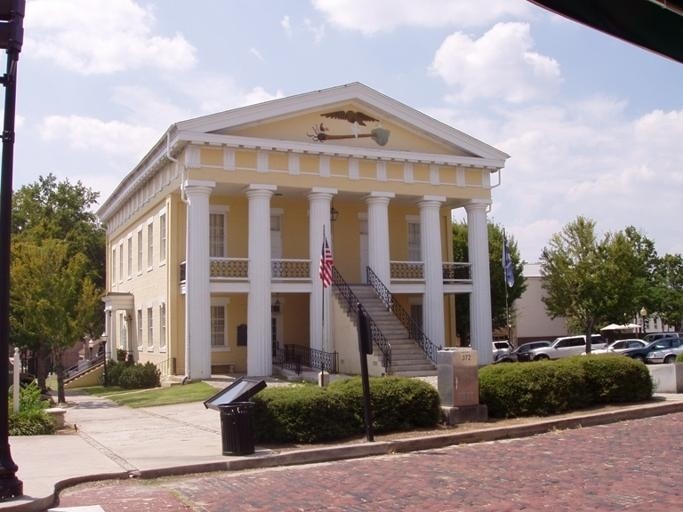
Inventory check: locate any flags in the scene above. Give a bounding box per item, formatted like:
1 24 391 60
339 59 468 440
502 236 515 288
317 237 335 289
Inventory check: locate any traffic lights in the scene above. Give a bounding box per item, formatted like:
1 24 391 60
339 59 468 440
0 0 16 47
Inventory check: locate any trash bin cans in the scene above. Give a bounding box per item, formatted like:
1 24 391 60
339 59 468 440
217 402 256 456
285 344 295 361
516 352 531 362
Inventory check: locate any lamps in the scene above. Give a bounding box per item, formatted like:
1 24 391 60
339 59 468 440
330 206 338 221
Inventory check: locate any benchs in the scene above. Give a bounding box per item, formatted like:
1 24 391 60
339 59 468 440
39 406 67 431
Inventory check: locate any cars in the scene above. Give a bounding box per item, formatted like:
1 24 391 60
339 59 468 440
7 367 37 399
489 331 682 367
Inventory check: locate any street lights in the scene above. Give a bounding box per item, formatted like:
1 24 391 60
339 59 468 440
640 304 648 333
86 337 95 367
98 330 110 386
